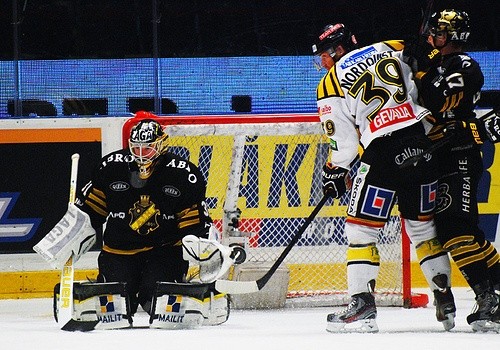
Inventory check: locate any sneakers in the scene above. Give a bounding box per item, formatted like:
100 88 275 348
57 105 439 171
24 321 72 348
432 274 456 331
327 280 378 333
467 296 500 334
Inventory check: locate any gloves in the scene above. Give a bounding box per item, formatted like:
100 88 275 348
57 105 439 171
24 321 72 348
321 163 348 198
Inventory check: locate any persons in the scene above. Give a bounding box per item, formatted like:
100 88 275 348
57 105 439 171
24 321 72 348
33 116 236 331
310 10 500 333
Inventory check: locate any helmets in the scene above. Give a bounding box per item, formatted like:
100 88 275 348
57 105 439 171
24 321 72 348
128 119 169 165
312 24 357 57
422 8 471 42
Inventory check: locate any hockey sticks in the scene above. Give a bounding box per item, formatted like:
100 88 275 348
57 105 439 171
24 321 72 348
477 105 500 121
58 154 102 332
215 190 332 294
